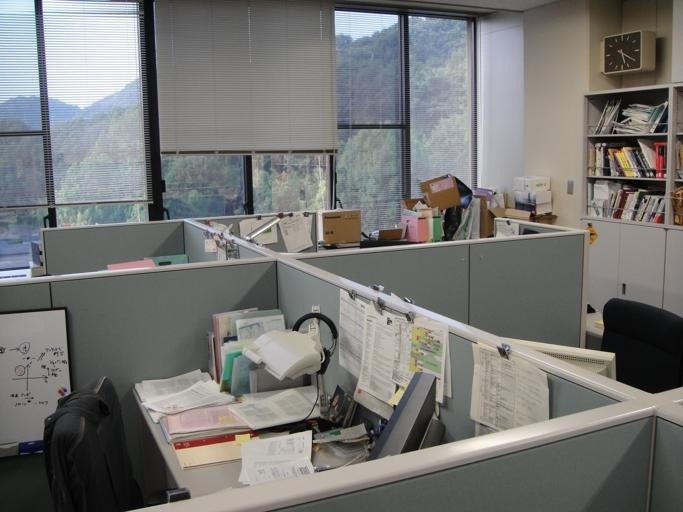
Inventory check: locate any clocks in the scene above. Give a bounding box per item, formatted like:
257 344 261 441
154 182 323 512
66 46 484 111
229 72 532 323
600 29 657 75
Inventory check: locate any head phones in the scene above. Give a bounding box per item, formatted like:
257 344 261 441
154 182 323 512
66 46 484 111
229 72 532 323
292 312 338 375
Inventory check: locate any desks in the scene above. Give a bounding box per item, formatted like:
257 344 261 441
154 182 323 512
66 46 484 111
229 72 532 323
133 367 424 501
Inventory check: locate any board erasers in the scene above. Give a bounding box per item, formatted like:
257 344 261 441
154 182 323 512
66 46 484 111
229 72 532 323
18 440 44 455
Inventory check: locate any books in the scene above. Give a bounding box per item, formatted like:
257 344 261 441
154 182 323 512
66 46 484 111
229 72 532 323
131 303 319 470
586 95 682 227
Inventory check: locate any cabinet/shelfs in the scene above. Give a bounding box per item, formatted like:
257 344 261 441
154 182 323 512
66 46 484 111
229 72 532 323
586 81 682 320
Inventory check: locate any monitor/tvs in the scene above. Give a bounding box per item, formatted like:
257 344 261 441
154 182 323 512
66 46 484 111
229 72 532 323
363 371 447 462
512 339 617 382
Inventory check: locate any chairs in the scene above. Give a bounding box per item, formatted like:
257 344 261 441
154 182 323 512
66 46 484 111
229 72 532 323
600 298 683 390
44 377 147 512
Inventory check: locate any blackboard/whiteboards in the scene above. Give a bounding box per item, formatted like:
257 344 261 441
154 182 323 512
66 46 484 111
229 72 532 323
0 306 71 458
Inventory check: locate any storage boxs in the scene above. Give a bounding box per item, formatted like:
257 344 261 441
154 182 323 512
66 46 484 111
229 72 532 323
320 173 553 245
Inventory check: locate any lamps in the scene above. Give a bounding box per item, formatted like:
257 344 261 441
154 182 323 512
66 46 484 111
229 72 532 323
244 311 337 443
